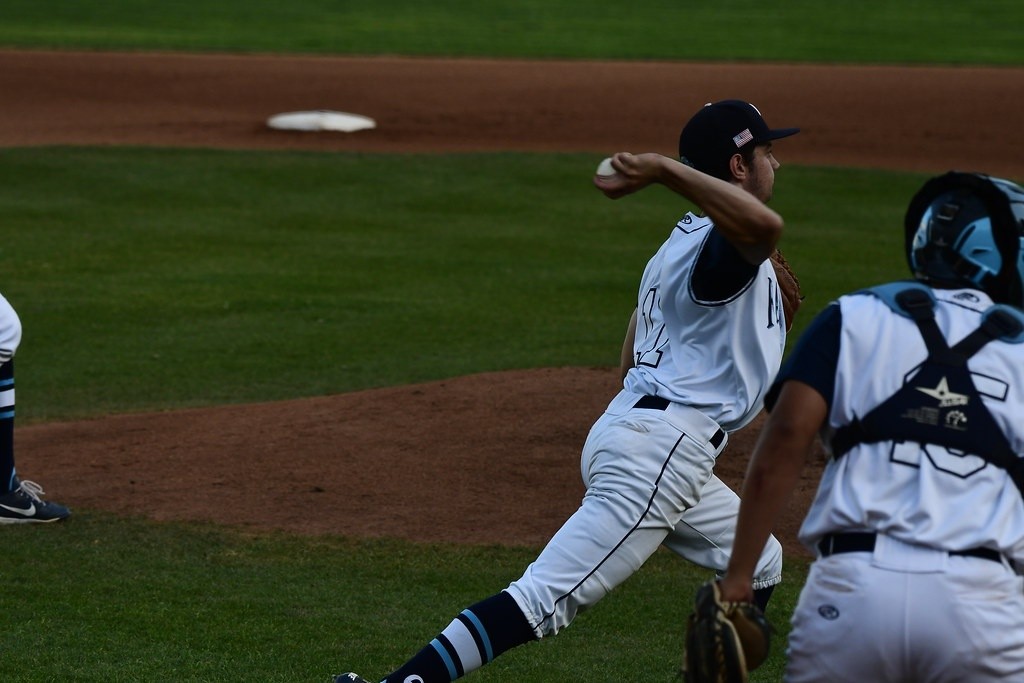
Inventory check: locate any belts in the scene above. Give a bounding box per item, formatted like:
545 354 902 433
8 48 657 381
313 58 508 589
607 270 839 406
819 531 1015 569
633 395 729 459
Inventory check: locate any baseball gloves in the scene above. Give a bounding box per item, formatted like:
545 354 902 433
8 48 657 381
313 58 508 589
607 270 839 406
684 576 772 683
769 248 803 334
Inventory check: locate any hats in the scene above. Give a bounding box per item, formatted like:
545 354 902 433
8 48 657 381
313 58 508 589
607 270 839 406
678 99 801 171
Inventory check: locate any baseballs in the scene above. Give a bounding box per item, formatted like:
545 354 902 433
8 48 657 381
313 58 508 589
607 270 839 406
597 156 624 181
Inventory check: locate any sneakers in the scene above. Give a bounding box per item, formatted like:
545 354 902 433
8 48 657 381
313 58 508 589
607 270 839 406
0 475 71 524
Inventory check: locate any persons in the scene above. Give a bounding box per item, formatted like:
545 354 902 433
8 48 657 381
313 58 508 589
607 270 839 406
0 292 70 524
684 167 1024 683
328 100 803 683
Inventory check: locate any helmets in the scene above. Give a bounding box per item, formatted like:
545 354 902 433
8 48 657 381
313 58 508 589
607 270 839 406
904 170 1024 308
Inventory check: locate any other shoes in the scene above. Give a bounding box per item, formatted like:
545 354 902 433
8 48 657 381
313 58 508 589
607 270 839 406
334 671 424 683
